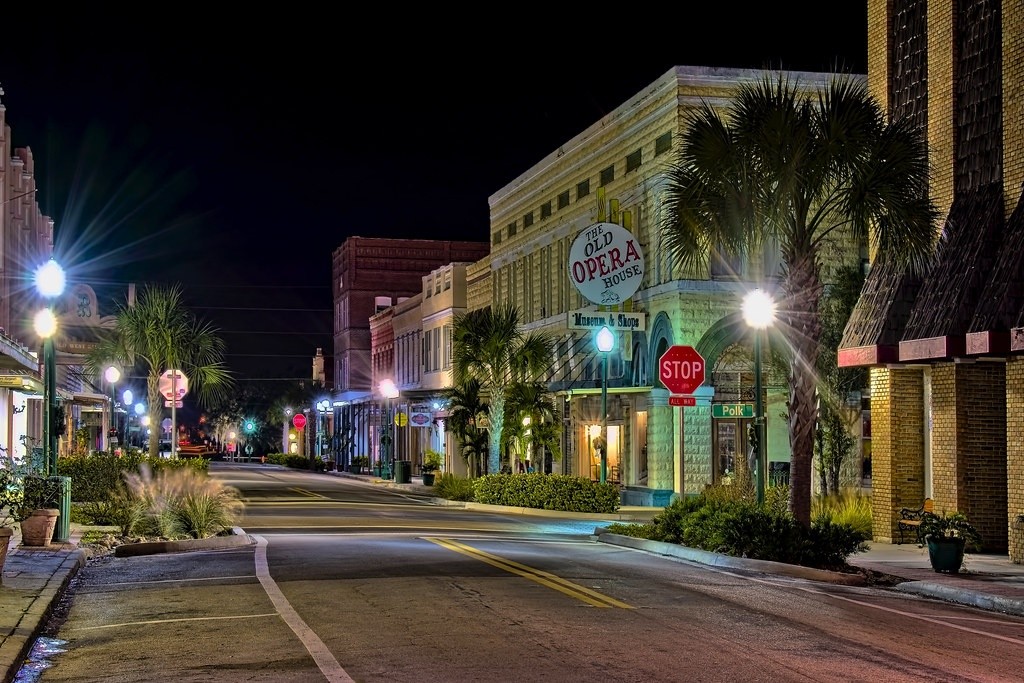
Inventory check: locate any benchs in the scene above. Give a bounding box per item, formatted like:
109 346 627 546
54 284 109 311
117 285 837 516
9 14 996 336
897 497 931 548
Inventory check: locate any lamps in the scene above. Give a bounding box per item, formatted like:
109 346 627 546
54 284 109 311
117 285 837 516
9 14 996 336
13 398 26 414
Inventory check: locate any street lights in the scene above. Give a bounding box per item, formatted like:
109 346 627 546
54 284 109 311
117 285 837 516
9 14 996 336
105 366 121 431
32 258 67 478
32 308 59 477
322 400 329 438
594 325 616 488
380 379 395 480
742 288 779 506
246 422 253 463
122 390 133 450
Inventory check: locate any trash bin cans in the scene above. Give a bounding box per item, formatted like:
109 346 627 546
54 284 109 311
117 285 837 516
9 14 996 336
394 460 412 483
23 474 72 543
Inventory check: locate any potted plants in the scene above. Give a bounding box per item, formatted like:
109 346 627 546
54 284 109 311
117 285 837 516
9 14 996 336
373 460 384 476
326 455 336 471
922 507 982 574
420 460 439 487
0 445 20 578
349 456 361 473
17 435 60 545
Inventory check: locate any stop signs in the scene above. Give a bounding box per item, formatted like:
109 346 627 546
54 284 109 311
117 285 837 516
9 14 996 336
659 344 706 396
292 414 306 429
